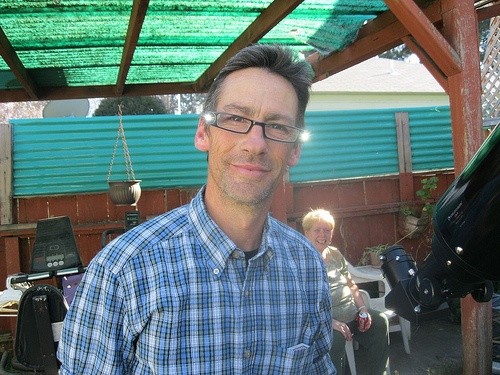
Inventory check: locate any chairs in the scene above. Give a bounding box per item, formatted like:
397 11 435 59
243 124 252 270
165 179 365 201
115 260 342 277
344 257 411 375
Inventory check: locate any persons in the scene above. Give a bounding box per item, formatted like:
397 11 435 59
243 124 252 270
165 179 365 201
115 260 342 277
56 44 336 375
301 208 391 375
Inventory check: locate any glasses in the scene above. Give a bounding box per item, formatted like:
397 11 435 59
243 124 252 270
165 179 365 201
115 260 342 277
213 111 298 143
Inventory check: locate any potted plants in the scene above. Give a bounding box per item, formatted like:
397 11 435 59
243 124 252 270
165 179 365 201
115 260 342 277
399 175 439 239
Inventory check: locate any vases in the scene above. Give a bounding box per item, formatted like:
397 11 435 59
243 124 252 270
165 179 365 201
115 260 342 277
369 252 384 269
109 179 142 207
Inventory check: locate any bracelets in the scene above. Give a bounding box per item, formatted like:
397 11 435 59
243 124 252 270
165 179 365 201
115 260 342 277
357 305 366 313
